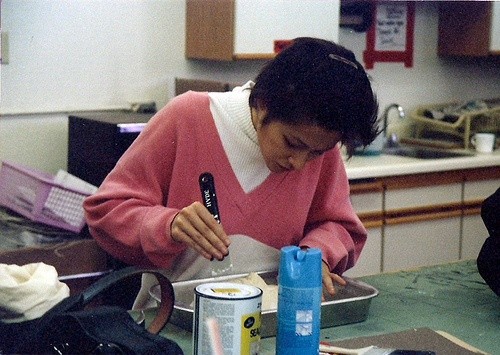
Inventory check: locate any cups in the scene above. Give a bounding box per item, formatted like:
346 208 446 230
471 133 496 153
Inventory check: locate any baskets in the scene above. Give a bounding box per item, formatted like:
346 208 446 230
0 161 98 234
409 100 500 149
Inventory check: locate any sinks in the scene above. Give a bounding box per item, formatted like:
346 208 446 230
381 146 477 160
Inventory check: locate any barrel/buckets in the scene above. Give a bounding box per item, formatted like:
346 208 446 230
191 282 264 355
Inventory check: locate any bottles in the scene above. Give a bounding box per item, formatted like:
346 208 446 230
274 245 323 355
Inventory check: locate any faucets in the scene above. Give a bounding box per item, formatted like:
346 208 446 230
383 102 406 147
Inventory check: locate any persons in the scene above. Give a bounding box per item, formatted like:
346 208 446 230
475 185 500 300
80 37 390 304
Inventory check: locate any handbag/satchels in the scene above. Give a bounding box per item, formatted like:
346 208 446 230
0 267 182 355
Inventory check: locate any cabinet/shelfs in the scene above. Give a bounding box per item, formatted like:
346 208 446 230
342 166 500 280
437 0 500 58
186 0 340 62
